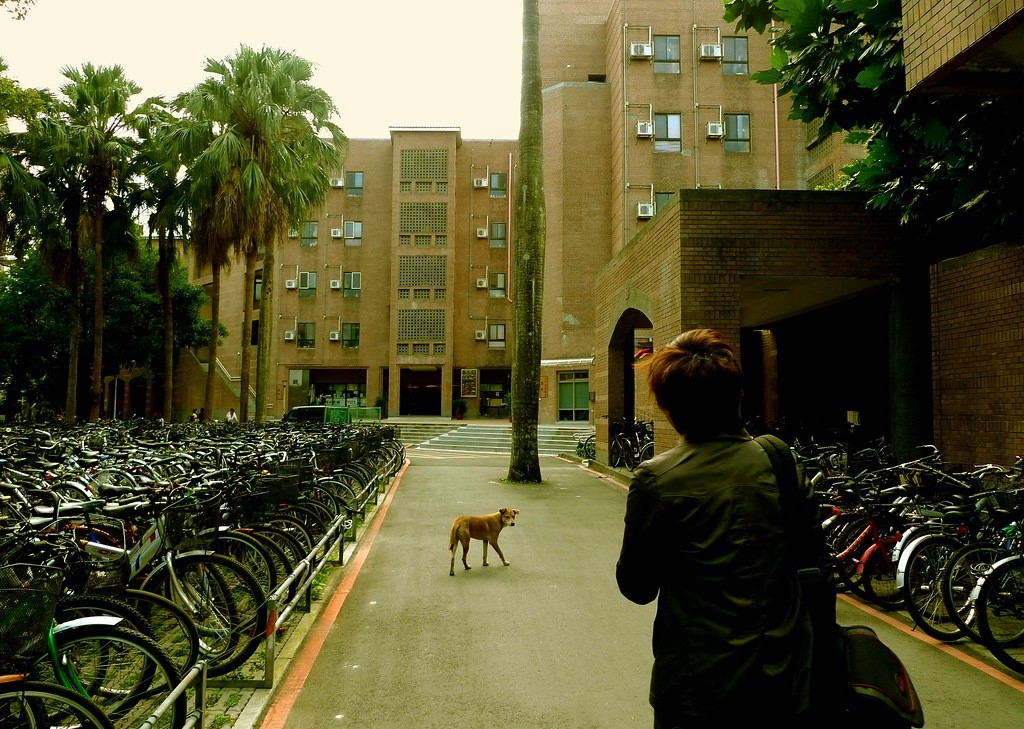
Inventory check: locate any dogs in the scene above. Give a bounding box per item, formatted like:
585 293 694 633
448 507 520 576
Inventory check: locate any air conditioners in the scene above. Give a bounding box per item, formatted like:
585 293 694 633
284 331 295 340
329 178 344 188
636 204 653 217
629 44 652 59
475 330 485 340
329 280 342 289
476 278 487 288
285 280 297 289
635 123 653 137
287 228 298 238
474 178 487 188
477 228 487 238
706 124 723 138
329 332 339 341
330 228 343 238
699 45 721 60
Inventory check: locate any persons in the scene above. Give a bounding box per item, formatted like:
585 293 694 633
225 408 238 422
197 408 205 424
615 327 834 729
191 408 199 422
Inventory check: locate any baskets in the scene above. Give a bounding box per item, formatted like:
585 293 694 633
816 448 1024 516
0 427 403 658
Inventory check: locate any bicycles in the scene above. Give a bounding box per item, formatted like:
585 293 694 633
744 419 1024 678
584 416 655 472
0 413 409 729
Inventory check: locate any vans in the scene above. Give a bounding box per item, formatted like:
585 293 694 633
281 404 352 422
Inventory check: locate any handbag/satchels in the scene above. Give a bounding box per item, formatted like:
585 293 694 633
816 624 923 729
